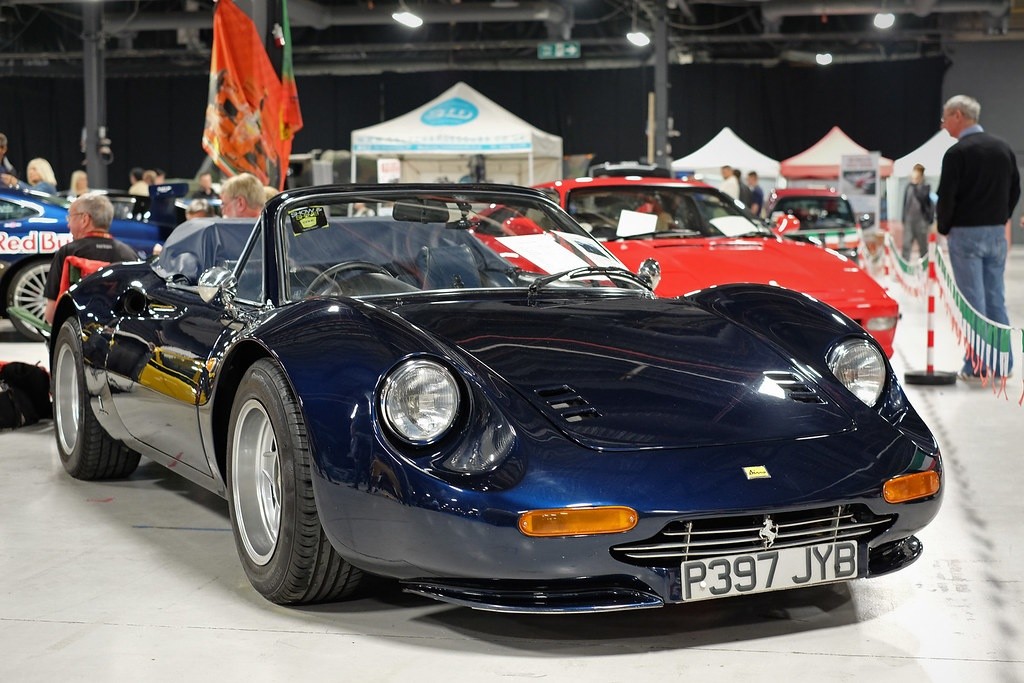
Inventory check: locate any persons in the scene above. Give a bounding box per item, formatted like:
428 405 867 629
935 95 1021 380
129 167 165 196
66 170 108 203
42 193 140 325
354 202 375 217
191 172 220 199
0 133 19 178
186 198 214 221
1 158 57 196
219 173 279 217
902 164 930 271
719 166 763 218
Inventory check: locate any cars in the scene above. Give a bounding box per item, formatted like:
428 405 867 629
466 176 899 361
754 186 874 271
0 187 163 341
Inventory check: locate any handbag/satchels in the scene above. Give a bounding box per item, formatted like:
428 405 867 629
0 361 53 428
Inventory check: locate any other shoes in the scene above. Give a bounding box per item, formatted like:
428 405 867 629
957 370 990 382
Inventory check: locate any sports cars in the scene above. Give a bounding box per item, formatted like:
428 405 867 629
49 183 947 610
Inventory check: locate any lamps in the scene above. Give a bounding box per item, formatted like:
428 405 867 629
391 0 424 29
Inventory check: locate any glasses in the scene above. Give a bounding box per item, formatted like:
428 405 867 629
65 213 93 223
941 111 956 123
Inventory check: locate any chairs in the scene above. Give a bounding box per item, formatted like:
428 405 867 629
7 255 110 349
414 243 483 290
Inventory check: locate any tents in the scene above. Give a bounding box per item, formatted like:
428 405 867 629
351 82 563 187
894 128 959 223
780 126 894 230
671 127 780 218
